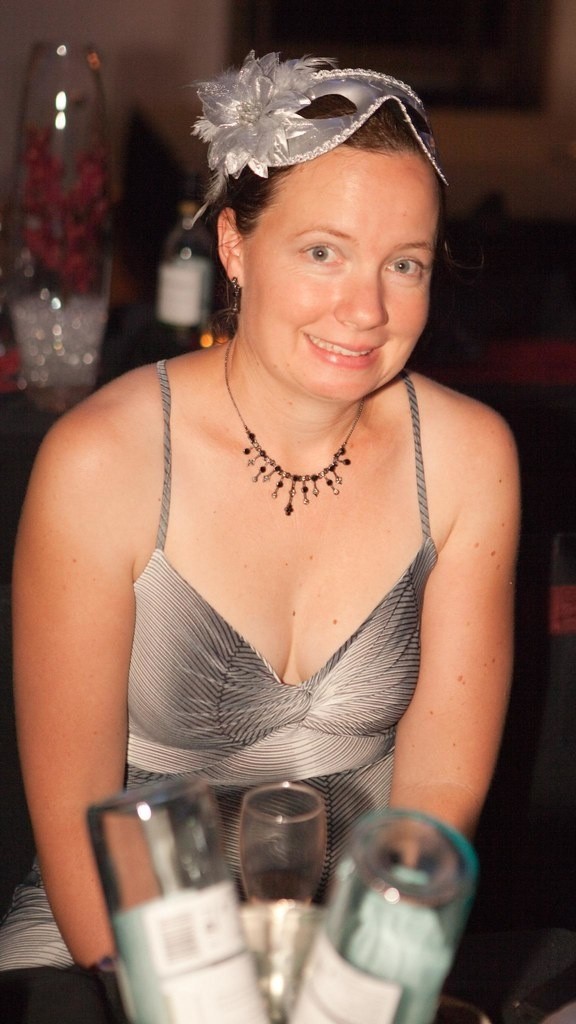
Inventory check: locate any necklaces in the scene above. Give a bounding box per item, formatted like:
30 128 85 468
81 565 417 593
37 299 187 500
224 341 364 516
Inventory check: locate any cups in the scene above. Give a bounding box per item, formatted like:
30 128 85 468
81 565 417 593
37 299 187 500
239 782 327 905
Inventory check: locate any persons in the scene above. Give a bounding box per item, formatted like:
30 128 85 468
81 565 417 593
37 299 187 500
0 51 521 973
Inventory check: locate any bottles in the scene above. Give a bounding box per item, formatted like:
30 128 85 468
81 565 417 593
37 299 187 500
86 773 478 1024
148 204 212 355
